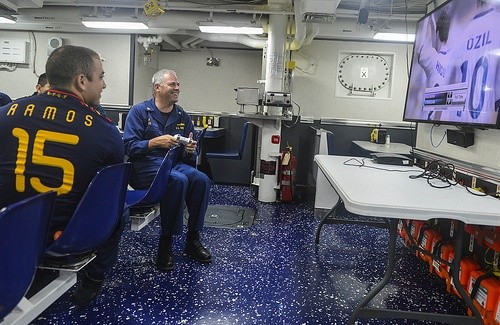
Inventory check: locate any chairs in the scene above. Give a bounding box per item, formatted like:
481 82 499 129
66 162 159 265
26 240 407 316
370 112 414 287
0 122 247 325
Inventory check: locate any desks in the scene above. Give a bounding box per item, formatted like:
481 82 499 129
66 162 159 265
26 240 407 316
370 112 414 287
193 127 225 171
353 141 414 161
313 154 500 325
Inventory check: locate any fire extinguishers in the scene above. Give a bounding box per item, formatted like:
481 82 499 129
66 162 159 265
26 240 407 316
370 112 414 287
278 141 298 202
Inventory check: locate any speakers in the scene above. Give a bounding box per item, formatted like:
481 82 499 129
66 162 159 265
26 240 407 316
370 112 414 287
446 130 474 148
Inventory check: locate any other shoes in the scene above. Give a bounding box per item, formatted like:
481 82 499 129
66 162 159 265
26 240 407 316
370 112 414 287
156 243 175 268
184 234 212 264
73 272 104 308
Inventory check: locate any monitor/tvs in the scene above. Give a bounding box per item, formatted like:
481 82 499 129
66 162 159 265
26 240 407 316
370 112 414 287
401 0 499 128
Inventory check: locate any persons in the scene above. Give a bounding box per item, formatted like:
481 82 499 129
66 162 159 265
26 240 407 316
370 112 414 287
0 46 125 309
122 69 212 270
417 0 500 125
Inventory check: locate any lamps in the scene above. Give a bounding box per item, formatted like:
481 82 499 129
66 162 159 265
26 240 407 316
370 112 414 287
81 8 148 30
196 11 264 35
369 21 416 42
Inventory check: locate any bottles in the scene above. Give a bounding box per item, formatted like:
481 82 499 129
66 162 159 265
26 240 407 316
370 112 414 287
385 135 390 144
